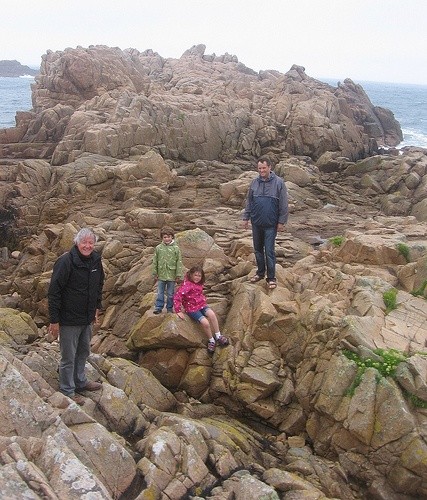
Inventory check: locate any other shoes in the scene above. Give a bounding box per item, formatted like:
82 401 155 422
207 341 215 352
214 335 228 345
69 396 85 406
167 311 173 314
153 310 162 314
82 380 102 391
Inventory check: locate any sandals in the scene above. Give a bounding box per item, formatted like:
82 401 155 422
265 277 277 289
250 274 264 282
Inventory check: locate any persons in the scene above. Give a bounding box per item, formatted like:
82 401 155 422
243 158 288 289
48 229 105 406
152 226 181 314
173 267 229 354
